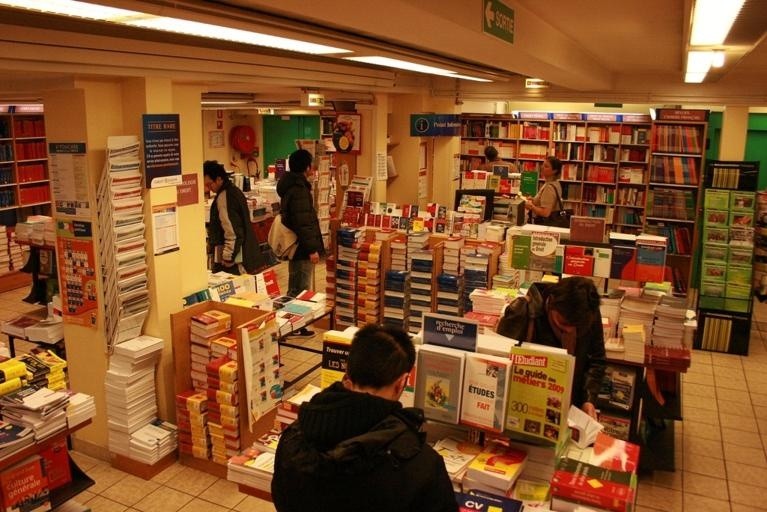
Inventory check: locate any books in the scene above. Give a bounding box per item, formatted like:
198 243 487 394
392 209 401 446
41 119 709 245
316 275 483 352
97 135 178 467
204 156 292 222
643 121 705 294
326 176 696 442
0 348 96 511
227 385 640 511
2 116 52 209
1 214 55 275
3 295 63 345
702 188 756 313
176 260 331 468
307 155 337 251
458 113 654 236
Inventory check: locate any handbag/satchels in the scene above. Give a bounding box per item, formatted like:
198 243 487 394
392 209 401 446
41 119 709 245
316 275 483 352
268 213 299 261
531 209 574 228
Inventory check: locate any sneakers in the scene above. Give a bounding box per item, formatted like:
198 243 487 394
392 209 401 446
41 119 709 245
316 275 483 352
288 326 315 337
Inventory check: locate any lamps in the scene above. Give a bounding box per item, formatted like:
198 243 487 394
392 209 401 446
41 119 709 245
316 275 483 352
680 0 766 85
346 55 497 82
0 0 353 55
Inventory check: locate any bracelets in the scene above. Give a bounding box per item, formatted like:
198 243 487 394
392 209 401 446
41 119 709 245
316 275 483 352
529 203 535 210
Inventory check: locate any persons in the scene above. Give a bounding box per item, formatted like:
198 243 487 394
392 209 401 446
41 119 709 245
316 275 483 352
525 157 574 228
496 276 608 422
203 160 264 276
270 321 460 512
268 149 324 340
476 146 518 173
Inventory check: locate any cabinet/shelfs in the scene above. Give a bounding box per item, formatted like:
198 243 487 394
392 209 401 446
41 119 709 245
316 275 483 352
328 216 502 340
95 147 149 356
693 157 761 358
0 111 52 294
461 117 710 290
597 352 683 477
167 298 285 480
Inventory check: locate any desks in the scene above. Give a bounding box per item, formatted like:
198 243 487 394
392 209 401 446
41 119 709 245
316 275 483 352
0 418 93 512
273 308 335 392
0 331 65 360
